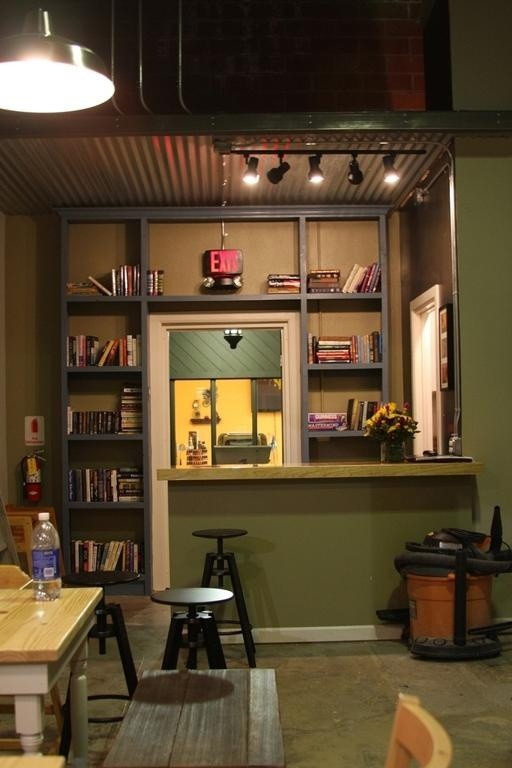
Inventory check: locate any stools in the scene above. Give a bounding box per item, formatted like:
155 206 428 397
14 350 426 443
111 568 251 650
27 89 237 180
189 528 257 668
58 570 142 755
152 588 235 670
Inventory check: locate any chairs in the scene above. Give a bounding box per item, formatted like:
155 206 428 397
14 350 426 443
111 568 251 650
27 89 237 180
0 565 37 590
385 693 454 768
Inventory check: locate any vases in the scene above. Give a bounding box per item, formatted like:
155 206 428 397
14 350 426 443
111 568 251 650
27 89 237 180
380 443 407 462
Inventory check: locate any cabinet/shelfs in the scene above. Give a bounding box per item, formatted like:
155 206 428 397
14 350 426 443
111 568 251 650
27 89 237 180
51 203 388 597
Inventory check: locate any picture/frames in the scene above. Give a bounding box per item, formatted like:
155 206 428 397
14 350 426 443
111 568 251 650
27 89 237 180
439 303 455 392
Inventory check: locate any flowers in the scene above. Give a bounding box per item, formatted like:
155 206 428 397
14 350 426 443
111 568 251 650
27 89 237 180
362 400 420 441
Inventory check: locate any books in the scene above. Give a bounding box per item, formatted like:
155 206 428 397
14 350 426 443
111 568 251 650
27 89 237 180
66 263 142 297
70 534 143 575
66 382 143 436
306 261 382 294
66 464 145 504
307 328 383 364
266 272 301 294
146 269 165 296
306 395 383 433
65 331 142 367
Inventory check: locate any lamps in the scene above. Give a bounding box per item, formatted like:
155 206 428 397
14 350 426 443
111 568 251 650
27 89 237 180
243 145 400 189
0 0 116 114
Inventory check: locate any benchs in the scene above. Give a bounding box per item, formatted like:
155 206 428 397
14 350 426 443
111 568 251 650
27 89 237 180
103 667 285 768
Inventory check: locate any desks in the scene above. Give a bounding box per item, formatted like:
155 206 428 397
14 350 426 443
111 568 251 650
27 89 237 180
1 586 105 767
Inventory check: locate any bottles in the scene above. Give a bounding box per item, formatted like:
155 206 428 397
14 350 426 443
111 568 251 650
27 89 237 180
30 512 62 601
